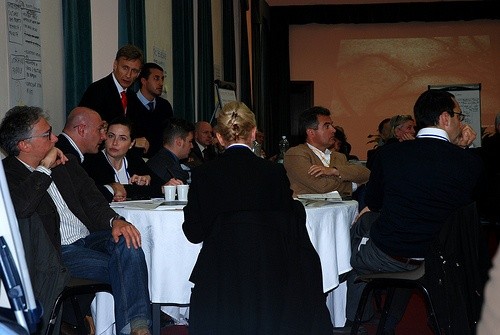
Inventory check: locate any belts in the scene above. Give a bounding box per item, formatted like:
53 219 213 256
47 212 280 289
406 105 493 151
388 255 422 265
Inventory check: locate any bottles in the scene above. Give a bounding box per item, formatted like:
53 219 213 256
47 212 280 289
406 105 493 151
279 135 288 162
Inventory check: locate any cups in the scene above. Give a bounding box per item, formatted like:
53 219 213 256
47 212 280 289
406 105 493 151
177 184 189 200
164 186 176 200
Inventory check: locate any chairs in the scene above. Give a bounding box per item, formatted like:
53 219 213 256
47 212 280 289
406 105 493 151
351 195 484 335
19 212 114 335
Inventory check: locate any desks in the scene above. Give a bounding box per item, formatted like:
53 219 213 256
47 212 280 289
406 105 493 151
92 195 359 335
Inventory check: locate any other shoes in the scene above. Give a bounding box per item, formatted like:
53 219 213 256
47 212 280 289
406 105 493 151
332 320 367 335
361 315 381 325
130 327 152 335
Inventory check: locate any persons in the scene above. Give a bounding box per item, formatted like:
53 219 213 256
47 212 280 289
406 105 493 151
183 118 225 162
56 107 174 327
333 89 476 335
327 128 359 161
378 114 416 139
285 106 370 197
182 100 294 286
136 63 174 170
0 106 152 335
147 119 194 198
78 46 149 155
482 114 500 138
97 118 161 201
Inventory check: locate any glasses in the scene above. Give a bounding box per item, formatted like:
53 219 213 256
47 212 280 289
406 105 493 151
438 111 465 122
17 127 52 146
74 124 106 133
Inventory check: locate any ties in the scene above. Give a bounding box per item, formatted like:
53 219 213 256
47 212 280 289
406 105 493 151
121 91 128 115
203 148 207 161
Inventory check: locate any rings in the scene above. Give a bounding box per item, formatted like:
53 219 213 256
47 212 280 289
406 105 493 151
141 180 146 182
